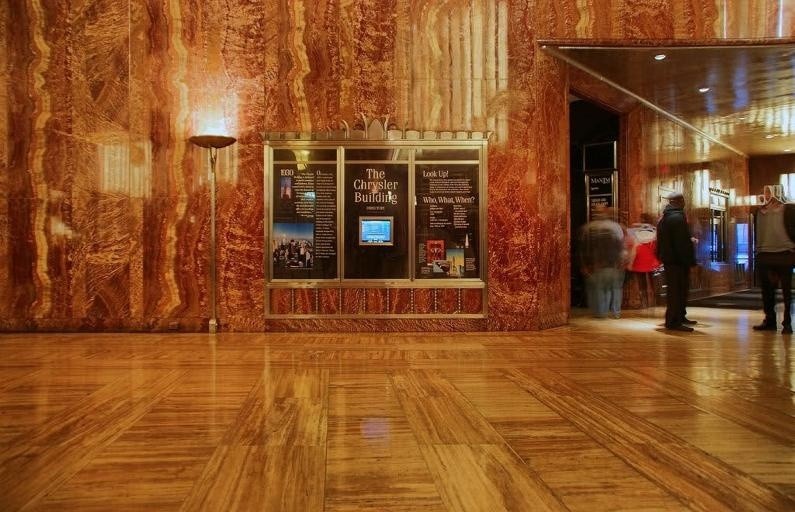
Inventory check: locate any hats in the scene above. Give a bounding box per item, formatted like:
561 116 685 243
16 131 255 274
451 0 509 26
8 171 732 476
667 191 684 202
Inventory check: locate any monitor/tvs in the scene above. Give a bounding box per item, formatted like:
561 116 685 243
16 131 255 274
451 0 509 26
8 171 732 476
358 216 396 248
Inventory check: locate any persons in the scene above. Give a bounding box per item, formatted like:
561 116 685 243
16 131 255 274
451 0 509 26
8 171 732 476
753 185 795 335
578 202 627 319
297 239 306 268
656 191 700 333
625 212 662 308
287 239 295 260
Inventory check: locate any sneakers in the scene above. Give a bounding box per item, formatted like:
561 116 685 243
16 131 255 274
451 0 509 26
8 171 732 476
781 320 793 334
753 317 777 331
665 324 694 335
681 316 697 326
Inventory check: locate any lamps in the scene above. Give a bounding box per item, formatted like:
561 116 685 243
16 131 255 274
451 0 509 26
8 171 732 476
188 134 237 334
